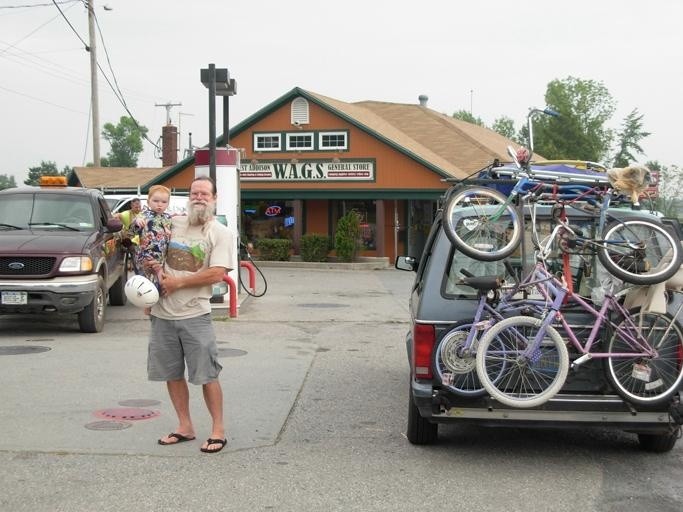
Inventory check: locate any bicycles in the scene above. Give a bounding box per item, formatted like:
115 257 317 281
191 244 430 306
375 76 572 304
442 106 683 284
515 234 683 406
477 271 570 407
431 266 564 399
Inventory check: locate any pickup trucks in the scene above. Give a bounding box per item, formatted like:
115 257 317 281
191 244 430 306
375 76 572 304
0 174 130 334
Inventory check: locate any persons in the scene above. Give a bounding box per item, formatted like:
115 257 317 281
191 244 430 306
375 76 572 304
114 198 141 245
129 185 172 316
144 177 235 453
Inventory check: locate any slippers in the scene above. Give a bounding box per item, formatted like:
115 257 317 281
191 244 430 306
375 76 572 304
157 432 196 446
200 437 227 454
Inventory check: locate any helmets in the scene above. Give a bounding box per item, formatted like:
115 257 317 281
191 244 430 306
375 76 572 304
124 274 159 309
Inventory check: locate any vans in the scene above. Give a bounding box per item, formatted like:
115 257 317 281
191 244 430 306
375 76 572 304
103 194 189 215
394 201 683 452
